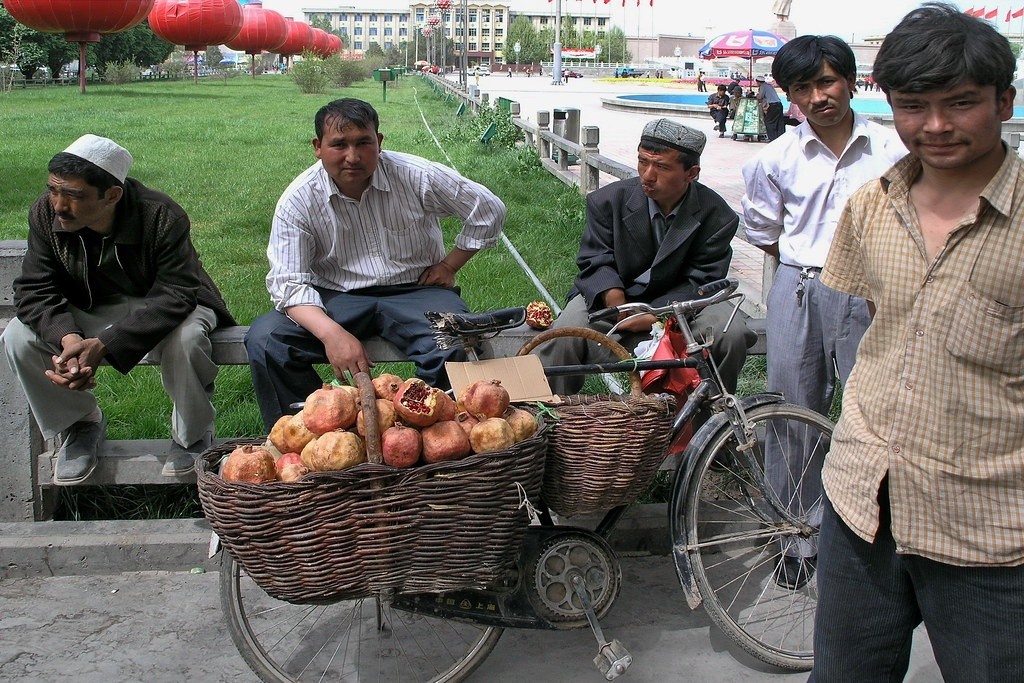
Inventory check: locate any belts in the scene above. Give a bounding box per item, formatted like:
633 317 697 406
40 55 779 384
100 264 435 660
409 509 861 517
769 103 779 105
785 265 823 272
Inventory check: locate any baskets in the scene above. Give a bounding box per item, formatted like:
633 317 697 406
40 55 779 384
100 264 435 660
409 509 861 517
519 327 678 519
194 374 549 605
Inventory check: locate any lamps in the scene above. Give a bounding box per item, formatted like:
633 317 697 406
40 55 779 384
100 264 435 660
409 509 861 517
420 72 452 103
480 122 497 145
456 102 465 118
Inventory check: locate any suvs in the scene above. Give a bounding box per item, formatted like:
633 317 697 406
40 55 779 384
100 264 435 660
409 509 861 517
764 73 774 82
479 64 487 71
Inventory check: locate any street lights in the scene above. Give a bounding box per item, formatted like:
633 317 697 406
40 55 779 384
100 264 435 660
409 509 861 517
413 25 418 71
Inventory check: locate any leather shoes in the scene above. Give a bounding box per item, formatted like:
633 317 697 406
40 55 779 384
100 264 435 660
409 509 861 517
53 410 107 486
161 418 214 477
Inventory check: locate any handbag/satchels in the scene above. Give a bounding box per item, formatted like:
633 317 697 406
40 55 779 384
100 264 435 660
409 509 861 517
564 78 565 81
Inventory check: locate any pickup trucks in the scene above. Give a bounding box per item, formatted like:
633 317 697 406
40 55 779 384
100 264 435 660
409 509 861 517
614 67 644 77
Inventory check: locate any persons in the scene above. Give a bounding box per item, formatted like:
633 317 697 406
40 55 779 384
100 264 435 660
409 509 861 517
535 118 759 472
474 54 664 87
741 35 910 589
856 73 881 92
2 133 239 486
271 62 287 74
697 69 805 141
805 1 1024 682
421 64 455 74
245 98 508 434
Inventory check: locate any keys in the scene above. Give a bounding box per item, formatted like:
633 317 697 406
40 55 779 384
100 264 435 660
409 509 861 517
795 288 805 307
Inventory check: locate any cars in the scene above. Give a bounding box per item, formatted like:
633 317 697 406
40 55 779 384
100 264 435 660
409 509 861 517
562 69 583 78
467 69 490 76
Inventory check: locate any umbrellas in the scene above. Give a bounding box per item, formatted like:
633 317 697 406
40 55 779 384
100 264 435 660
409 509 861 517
698 29 790 92
414 60 429 66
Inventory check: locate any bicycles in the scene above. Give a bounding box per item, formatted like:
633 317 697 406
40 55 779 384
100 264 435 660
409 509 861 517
208 278 838 683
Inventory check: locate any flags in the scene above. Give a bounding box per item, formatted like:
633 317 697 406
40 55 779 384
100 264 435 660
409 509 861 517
593 0 654 8
964 7 1024 22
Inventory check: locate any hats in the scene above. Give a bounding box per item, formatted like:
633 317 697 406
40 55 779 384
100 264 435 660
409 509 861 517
641 119 707 156
756 76 765 81
62 133 132 184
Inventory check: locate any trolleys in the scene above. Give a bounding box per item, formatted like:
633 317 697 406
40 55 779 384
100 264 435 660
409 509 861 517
731 98 769 142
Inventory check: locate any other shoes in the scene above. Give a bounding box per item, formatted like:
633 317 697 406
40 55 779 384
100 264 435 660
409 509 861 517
709 450 733 473
714 126 720 129
719 134 724 137
774 553 818 591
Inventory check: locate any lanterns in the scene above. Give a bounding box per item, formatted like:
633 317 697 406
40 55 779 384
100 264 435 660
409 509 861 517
2 0 244 91
326 31 344 55
225 3 289 78
309 25 330 63
273 16 317 72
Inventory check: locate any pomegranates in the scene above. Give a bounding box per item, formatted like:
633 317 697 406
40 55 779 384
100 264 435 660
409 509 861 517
526 301 553 329
223 382 364 484
372 374 537 467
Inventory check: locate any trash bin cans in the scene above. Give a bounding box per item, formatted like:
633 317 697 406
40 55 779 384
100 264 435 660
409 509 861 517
553 107 580 166
